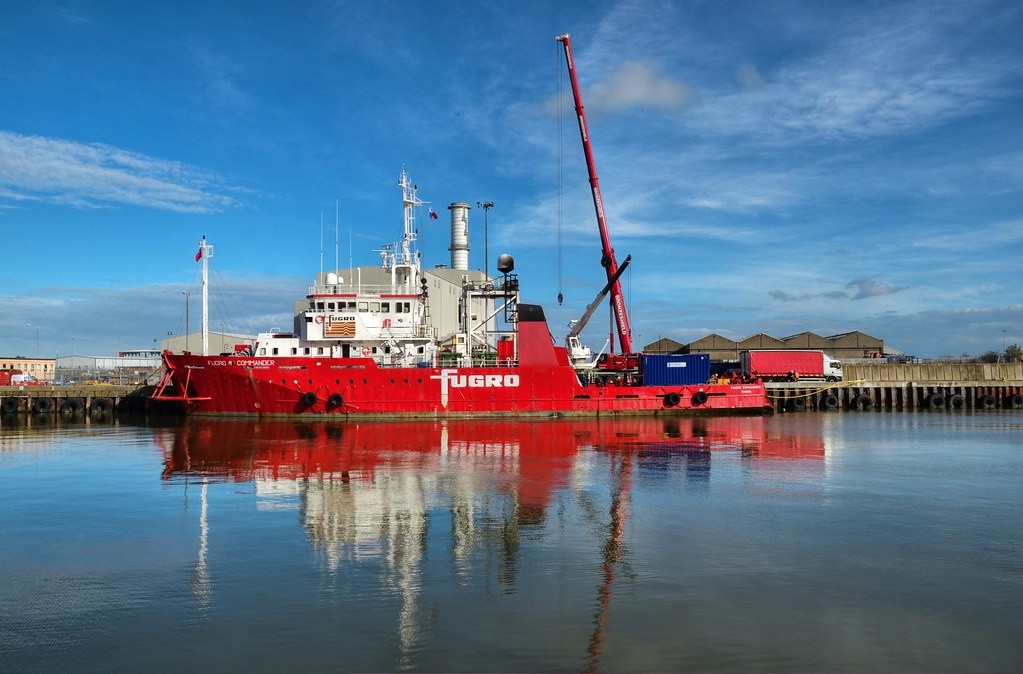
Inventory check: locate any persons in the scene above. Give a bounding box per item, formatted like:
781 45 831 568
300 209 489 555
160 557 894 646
788 370 799 382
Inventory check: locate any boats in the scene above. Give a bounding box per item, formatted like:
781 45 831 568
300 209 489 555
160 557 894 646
161 407 777 668
141 163 777 419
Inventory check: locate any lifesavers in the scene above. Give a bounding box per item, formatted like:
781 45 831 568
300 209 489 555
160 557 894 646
3 397 19 412
824 395 838 409
329 393 343 407
34 398 51 413
362 346 371 355
949 394 965 408
304 392 316 405
90 398 112 412
794 398 806 409
929 394 946 408
1009 394 1023 410
668 392 680 405
61 400 84 412
315 315 324 324
855 394 873 408
695 391 707 404
980 395 997 410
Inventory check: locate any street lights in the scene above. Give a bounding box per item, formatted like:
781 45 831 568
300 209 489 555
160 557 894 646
1002 329 1007 353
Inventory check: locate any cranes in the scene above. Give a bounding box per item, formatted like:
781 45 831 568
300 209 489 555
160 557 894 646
564 253 632 361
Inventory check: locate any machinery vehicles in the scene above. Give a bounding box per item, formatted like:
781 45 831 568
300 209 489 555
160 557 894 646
556 33 642 371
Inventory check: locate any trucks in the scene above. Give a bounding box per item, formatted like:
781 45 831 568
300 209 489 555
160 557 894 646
739 350 843 383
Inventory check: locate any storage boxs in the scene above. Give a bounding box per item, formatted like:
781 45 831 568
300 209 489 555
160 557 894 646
643 353 711 386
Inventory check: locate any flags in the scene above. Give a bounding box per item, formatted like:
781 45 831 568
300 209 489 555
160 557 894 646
428 206 439 221
195 251 201 262
